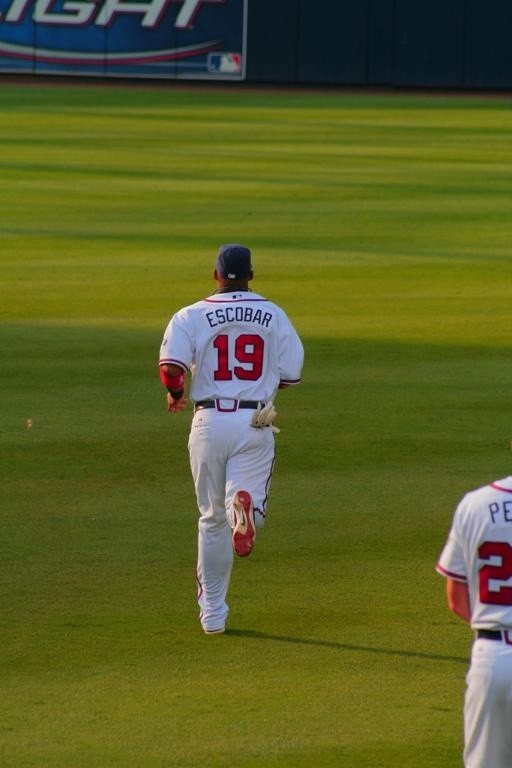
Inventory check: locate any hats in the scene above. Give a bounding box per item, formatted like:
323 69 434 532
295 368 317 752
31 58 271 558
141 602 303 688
215 241 251 280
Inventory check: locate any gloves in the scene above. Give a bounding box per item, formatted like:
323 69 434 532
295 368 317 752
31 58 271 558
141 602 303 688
252 402 282 436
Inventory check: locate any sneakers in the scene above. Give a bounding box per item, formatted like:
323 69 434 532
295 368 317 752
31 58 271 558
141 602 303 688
230 487 257 559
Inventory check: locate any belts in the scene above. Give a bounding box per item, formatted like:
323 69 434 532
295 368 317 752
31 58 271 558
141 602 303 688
474 627 502 642
195 399 265 410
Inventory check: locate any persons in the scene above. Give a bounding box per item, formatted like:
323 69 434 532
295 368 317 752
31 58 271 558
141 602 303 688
435 476 512 767
159 244 304 635
